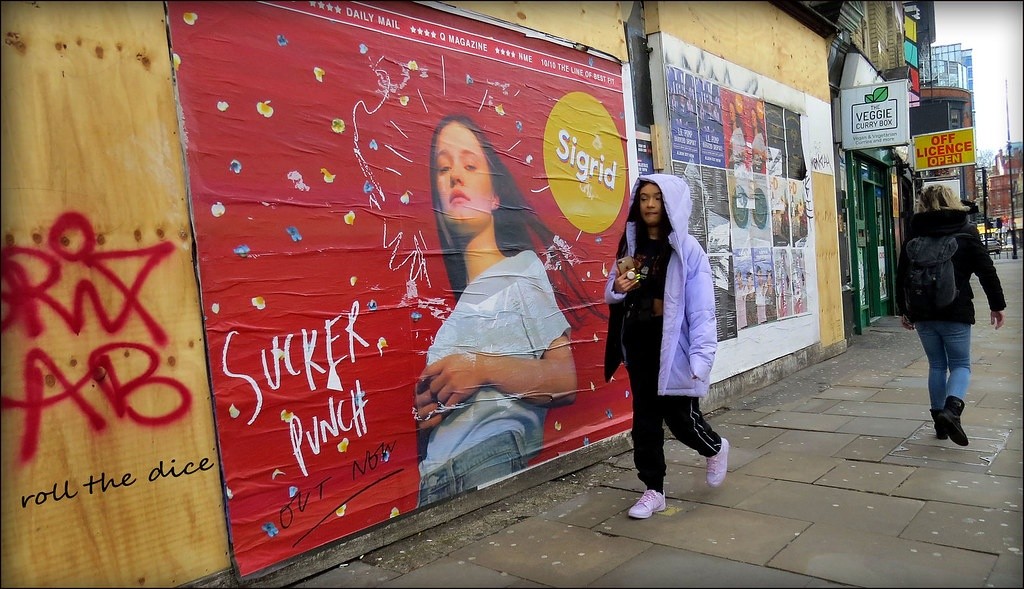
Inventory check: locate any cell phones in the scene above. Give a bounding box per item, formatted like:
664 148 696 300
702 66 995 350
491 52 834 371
617 257 641 291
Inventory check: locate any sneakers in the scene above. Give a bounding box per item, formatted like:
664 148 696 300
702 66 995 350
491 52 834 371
628 489 666 519
705 437 730 487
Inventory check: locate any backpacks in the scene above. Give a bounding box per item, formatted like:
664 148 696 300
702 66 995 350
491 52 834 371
907 230 977 309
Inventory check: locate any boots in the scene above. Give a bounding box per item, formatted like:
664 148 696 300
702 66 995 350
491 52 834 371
938 395 969 446
929 407 948 440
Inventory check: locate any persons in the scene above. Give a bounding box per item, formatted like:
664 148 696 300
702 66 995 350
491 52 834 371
603 173 729 518
750 108 767 173
736 270 755 300
895 184 1007 446
414 115 608 508
756 266 778 323
728 103 747 169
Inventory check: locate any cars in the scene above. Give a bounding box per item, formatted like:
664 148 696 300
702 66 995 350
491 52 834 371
982 238 1002 254
